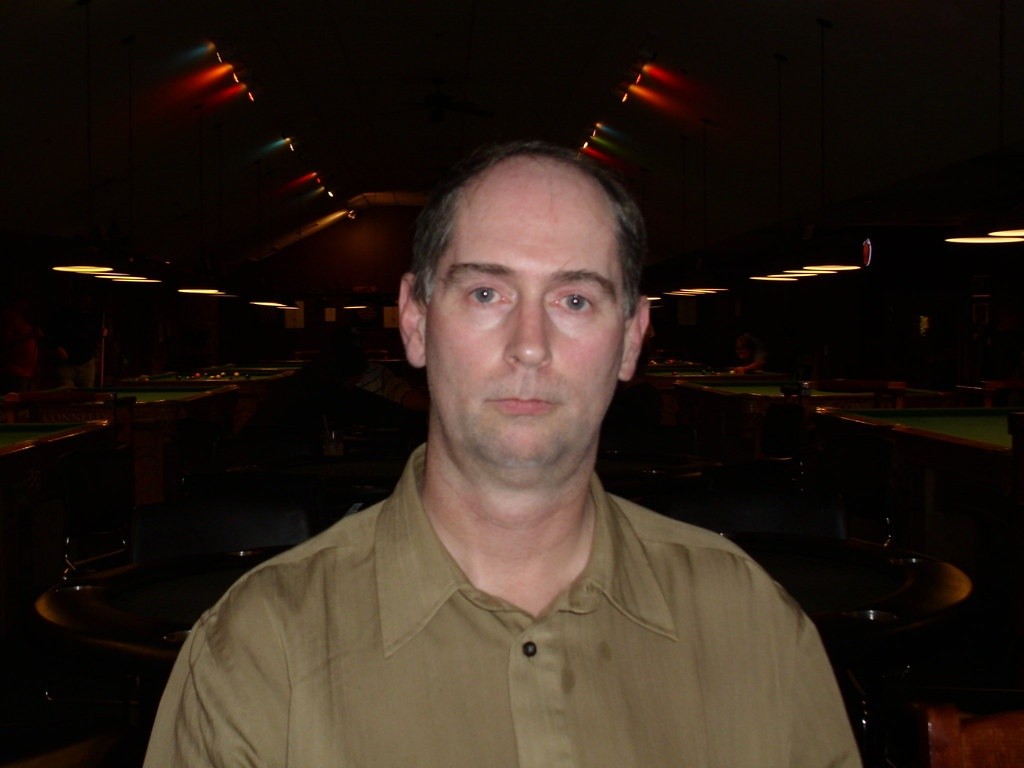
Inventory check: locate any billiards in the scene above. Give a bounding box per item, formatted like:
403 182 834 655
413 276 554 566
177 371 250 380
647 358 736 377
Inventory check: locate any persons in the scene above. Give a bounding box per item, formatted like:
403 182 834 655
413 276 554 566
0 291 46 392
144 140 865 768
733 334 797 377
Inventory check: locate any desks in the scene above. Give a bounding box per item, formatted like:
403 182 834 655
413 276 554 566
30 530 973 768
0 355 1024 646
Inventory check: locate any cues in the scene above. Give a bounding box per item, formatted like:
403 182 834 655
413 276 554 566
98 308 106 387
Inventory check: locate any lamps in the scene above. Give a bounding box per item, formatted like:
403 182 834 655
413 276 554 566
47 0 866 308
